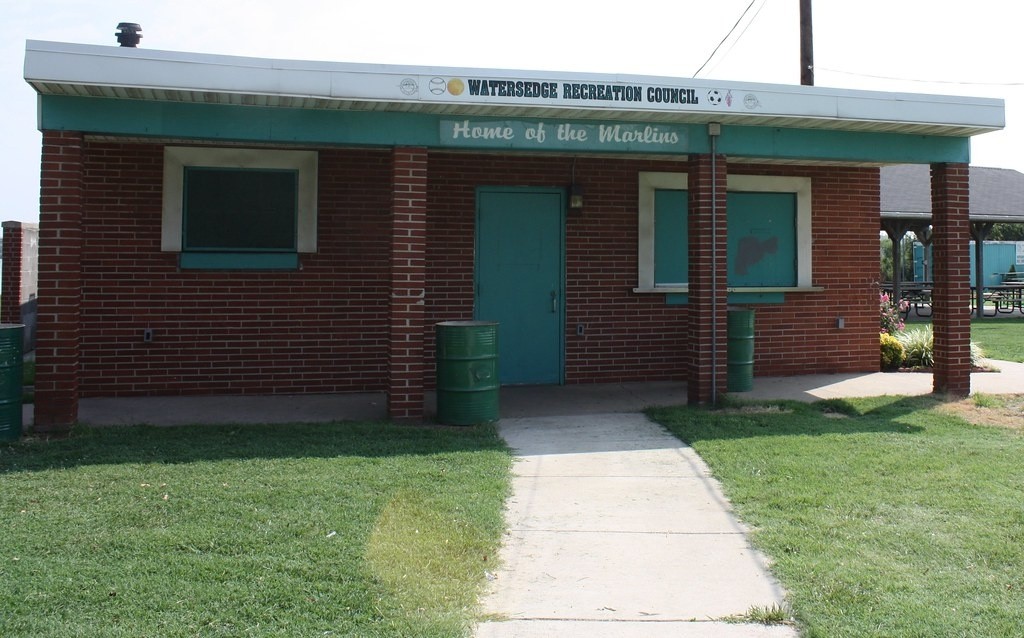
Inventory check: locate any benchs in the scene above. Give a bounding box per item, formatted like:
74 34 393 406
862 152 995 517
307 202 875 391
971 277 1024 303
881 287 933 304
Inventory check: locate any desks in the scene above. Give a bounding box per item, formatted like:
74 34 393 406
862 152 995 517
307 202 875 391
901 281 934 308
992 271 1024 292
879 281 904 307
999 281 1024 309
879 286 933 320
970 284 1024 317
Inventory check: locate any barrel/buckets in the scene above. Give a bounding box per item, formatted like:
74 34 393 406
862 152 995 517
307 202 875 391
724 306 755 392
435 320 502 426
0 323 25 442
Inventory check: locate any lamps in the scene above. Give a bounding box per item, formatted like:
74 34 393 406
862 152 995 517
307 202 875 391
565 175 585 219
